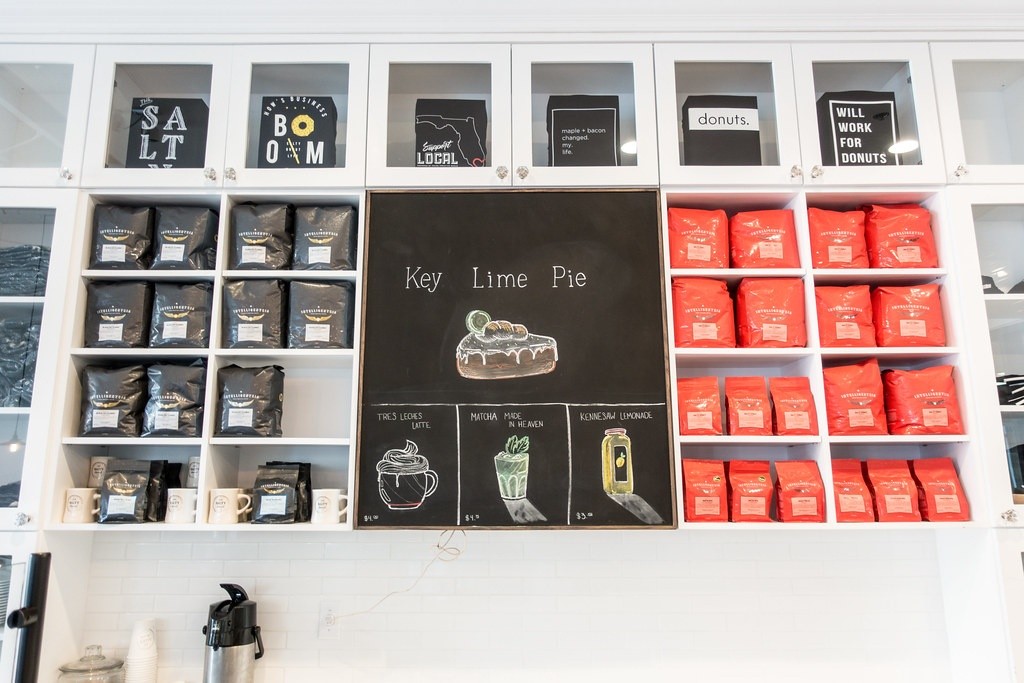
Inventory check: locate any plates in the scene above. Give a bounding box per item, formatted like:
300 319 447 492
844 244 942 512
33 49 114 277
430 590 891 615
0 570 12 628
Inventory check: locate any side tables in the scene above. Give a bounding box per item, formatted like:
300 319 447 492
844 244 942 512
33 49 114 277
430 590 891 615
972 195 1024 512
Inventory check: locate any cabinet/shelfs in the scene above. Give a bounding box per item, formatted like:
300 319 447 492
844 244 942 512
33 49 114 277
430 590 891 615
931 43 1024 177
656 47 939 186
86 33 366 192
372 42 660 195
51 190 363 534
0 191 76 531
0 38 105 190
659 188 970 529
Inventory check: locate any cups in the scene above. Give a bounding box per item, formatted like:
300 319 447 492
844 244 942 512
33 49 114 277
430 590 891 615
309 489 348 524
120 616 159 683
87 455 118 488
208 488 254 523
62 487 104 523
184 457 201 488
165 488 198 523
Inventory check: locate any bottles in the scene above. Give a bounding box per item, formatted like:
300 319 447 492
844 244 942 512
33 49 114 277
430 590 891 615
57 643 124 683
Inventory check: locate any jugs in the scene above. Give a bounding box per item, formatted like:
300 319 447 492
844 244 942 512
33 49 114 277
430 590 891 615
200 582 263 683
